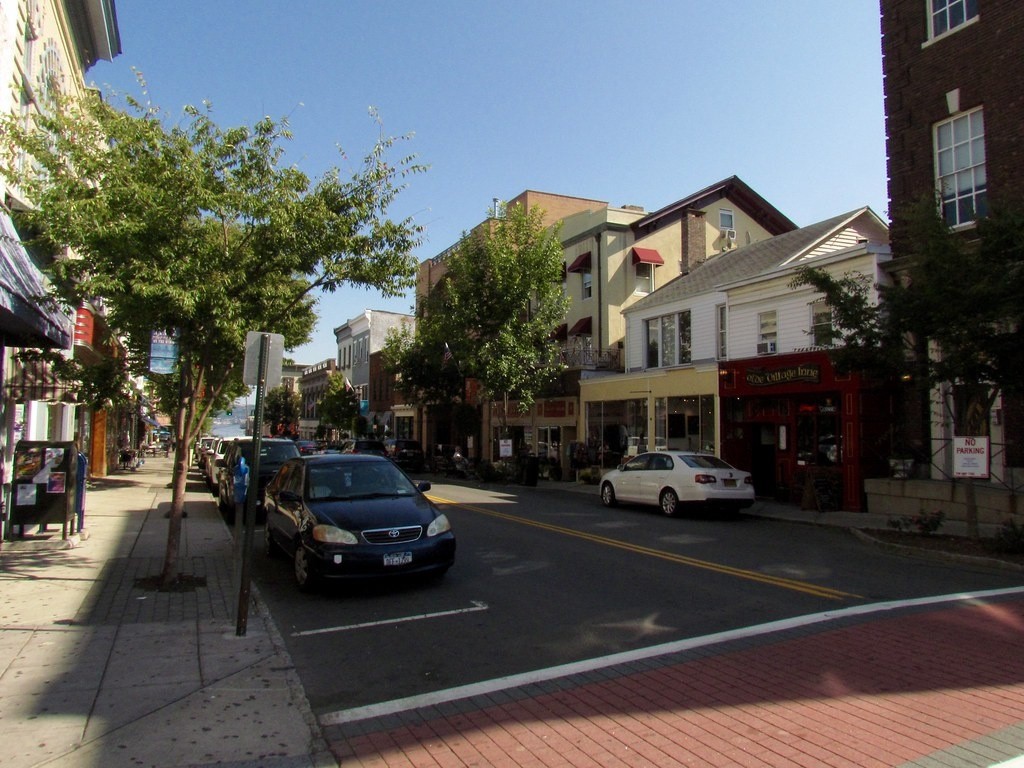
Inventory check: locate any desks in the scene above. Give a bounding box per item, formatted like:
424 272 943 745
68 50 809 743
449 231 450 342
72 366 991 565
116 450 132 470
146 447 162 457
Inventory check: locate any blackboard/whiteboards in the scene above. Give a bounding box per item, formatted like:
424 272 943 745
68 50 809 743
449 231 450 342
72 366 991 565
800 465 843 512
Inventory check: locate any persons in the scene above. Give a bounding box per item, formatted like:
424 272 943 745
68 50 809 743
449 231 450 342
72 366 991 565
366 468 409 494
155 435 159 443
517 443 562 482
554 336 582 365
171 435 177 451
573 434 609 467
453 446 468 469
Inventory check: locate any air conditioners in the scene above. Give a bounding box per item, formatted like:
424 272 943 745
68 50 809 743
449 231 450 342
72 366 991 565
756 342 776 356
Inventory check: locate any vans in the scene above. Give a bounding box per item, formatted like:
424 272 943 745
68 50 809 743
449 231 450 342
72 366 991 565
628 437 666 457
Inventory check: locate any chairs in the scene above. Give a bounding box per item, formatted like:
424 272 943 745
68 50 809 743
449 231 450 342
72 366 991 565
655 459 666 468
310 472 329 486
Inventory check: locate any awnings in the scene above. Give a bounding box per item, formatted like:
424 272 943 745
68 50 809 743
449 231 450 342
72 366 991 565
550 262 566 282
546 323 567 341
568 251 591 273
568 316 592 337
633 247 664 267
145 418 160 428
139 394 156 409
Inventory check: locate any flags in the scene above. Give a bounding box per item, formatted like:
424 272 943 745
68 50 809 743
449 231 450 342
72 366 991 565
345 378 351 392
441 345 452 371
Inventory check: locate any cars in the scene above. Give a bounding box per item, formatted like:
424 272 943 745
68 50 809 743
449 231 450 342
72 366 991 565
599 451 755 517
196 435 426 523
265 454 457 590
